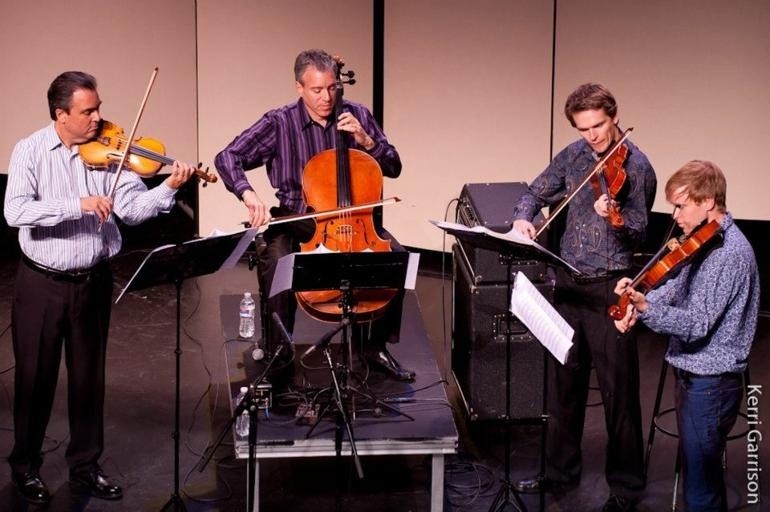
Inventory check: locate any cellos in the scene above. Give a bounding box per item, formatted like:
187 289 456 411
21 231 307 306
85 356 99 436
297 55 397 322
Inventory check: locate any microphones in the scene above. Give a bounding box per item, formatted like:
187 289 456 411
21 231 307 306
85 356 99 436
272 311 296 354
307 318 350 362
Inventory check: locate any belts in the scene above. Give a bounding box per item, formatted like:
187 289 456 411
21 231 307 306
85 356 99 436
566 272 622 285
22 254 103 282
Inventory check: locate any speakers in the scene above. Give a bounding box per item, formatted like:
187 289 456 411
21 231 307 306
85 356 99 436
456 182 548 284
450 244 557 426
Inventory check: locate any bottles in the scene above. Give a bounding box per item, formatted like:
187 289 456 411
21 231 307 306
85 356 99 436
238 291 256 341
229 387 257 436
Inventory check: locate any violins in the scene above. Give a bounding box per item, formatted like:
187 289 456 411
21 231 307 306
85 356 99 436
588 143 632 230
606 219 722 321
79 121 217 187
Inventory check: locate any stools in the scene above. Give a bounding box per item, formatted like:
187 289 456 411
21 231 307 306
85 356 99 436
646 335 763 512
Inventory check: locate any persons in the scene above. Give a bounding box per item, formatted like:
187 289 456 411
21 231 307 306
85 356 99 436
2 67 198 507
609 154 763 512
213 48 422 385
505 79 658 512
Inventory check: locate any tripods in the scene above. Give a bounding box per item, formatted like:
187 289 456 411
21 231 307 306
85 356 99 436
303 305 414 437
487 341 528 512
161 286 189 512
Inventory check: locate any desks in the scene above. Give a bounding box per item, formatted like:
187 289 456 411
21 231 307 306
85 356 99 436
217 288 460 509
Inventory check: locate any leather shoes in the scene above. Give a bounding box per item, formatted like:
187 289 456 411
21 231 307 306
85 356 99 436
513 472 579 493
68 468 123 499
11 471 49 503
369 349 415 381
603 494 633 512
